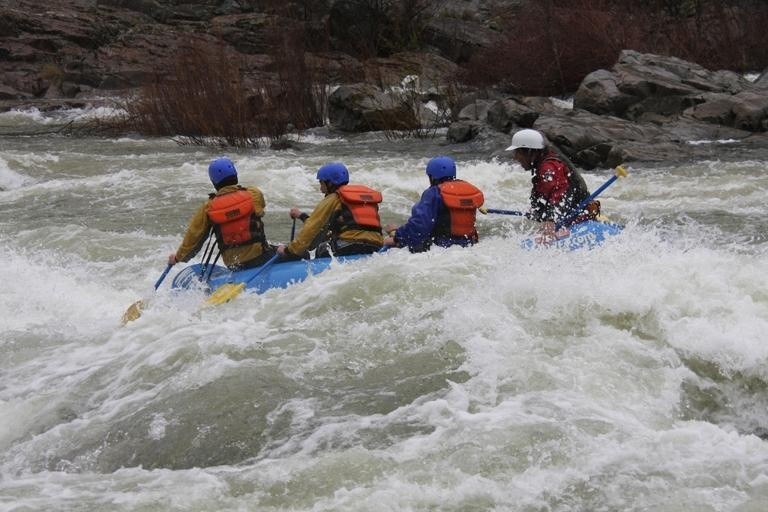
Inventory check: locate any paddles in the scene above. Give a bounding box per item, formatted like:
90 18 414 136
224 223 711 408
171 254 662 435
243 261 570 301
478 207 611 222
204 251 280 307
121 265 172 327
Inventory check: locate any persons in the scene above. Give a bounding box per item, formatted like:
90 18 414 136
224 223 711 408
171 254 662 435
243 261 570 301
275 162 384 260
504 127 600 246
383 154 486 253
167 156 302 272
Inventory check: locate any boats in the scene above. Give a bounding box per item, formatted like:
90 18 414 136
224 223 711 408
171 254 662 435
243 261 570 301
171 214 625 295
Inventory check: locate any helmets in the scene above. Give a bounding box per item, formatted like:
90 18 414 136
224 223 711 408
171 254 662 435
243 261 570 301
209 159 236 185
505 129 544 151
427 156 456 180
317 163 349 185
